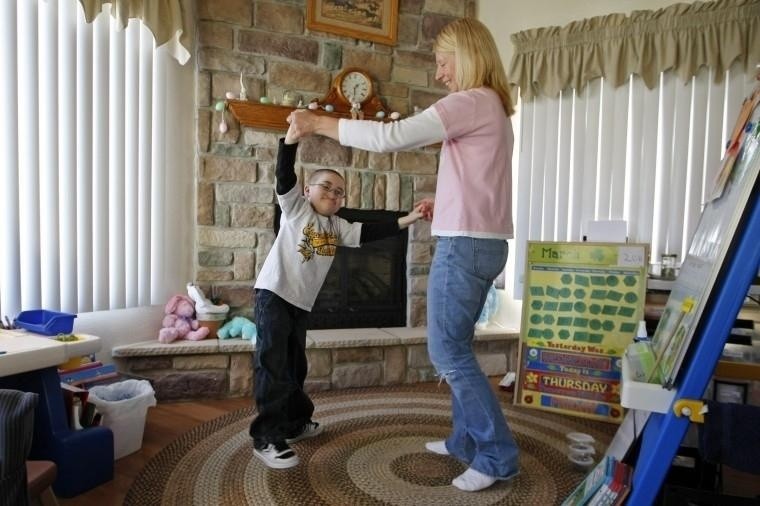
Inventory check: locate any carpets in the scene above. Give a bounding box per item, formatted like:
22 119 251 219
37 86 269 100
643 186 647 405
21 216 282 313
122 394 613 506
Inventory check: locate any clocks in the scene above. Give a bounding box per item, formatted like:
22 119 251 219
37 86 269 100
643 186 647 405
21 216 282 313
329 67 376 107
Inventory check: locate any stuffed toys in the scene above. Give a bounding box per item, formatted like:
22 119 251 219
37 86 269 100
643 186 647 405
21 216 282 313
215 313 258 342
185 281 231 316
155 293 210 345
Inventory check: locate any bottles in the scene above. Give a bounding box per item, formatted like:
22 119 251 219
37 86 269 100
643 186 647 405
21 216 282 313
661 254 677 280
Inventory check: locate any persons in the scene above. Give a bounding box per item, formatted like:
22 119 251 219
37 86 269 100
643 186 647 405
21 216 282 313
246 111 435 470
284 16 525 495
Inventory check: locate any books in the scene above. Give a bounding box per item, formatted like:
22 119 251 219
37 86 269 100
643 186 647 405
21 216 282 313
561 455 636 506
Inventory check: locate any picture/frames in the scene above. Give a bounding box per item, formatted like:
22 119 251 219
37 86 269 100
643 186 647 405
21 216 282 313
306 0 399 46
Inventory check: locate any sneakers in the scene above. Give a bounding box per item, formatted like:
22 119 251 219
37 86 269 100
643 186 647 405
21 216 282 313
252 439 301 470
285 421 323 444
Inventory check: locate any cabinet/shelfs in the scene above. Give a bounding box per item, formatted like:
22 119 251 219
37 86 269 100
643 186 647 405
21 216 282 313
646 275 760 363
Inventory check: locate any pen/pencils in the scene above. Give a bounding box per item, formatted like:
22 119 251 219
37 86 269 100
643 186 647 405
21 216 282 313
645 310 686 383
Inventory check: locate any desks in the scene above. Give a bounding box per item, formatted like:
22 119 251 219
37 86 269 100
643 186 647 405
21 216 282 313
1 329 114 497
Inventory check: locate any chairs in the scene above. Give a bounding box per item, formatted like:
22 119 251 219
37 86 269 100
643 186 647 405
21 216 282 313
1 387 62 506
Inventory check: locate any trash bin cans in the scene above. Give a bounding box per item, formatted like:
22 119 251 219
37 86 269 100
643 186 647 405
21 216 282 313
87 378 157 462
